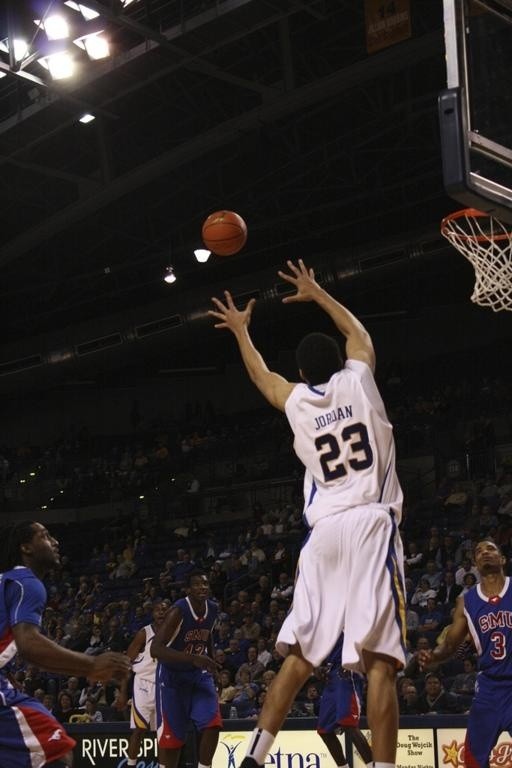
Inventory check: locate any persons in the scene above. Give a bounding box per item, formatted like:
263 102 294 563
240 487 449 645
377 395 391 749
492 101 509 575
316 644 375 767
1 520 134 768
116 596 170 768
418 538 512 767
207 259 408 768
151 572 226 768
1 377 509 720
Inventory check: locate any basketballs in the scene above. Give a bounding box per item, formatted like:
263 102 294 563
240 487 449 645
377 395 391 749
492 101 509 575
200 211 248 254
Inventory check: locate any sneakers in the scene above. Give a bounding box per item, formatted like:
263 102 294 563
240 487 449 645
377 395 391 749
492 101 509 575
238 756 264 768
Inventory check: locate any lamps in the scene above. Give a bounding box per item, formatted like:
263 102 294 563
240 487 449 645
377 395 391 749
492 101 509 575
162 232 177 288
0 0 115 81
192 216 211 263
